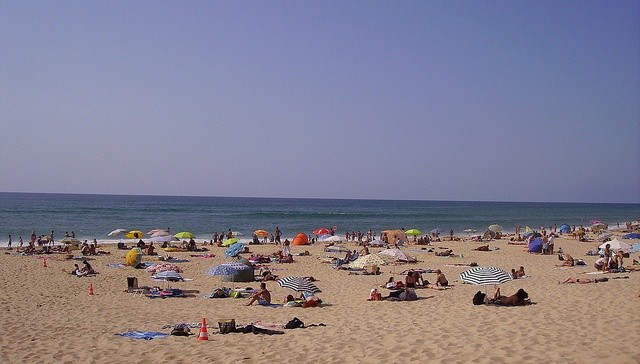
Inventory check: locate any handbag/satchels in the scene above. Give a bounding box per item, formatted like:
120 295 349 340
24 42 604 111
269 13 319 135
217 319 235 334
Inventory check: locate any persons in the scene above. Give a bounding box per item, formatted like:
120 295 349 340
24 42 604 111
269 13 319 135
518 234 521 240
315 234 319 242
283 239 290 255
92 238 98 246
594 258 603 271
80 260 95 273
187 238 195 250
547 233 555 256
359 245 369 254
336 250 357 271
556 254 573 267
38 235 51 245
80 244 89 255
579 224 585 242
217 238 224 247
385 277 397 289
487 233 492 242
489 288 531 307
162 242 168 247
7 234 12 249
31 231 36 244
311 237 314 243
65 231 69 237
146 241 156 255
432 233 442 242
572 224 577 239
623 253 630 258
472 245 500 251
275 227 282 246
249 230 260 244
539 224 561 235
18 237 23 247
90 243 96 255
586 228 590 232
381 227 407 248
248 250 293 263
406 271 416 287
299 290 322 307
81 239 88 247
72 263 79 274
602 244 612 271
610 250 624 272
518 267 525 277
448 229 454 241
620 219 639 238
434 269 449 286
187 238 197 250
159 289 183 297
308 235 311 240
413 270 425 288
258 264 272 280
472 245 490 251
434 250 453 256
511 268 518 279
49 229 54 246
345 229 376 246
329 228 334 235
218 231 224 241
541 233 547 255
562 277 598 284
243 283 270 306
421 280 452 291
418 235 430 245
71 231 74 238
395 238 400 247
226 228 232 238
212 232 219 243
270 234 274 242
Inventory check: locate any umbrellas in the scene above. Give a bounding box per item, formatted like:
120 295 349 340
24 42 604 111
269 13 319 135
591 223 608 231
407 229 423 235
108 229 128 237
223 236 239 245
488 225 503 231
529 237 542 252
561 223 570 234
379 248 416 262
253 229 268 236
150 271 185 292
124 247 142 267
596 232 614 240
225 243 246 256
175 232 197 238
525 225 532 233
206 265 238 289
128 230 143 240
225 261 247 289
524 232 541 237
598 238 630 251
61 238 77 253
291 232 308 245
313 227 328 235
278 276 320 299
348 253 395 291
148 230 179 242
460 266 511 296
